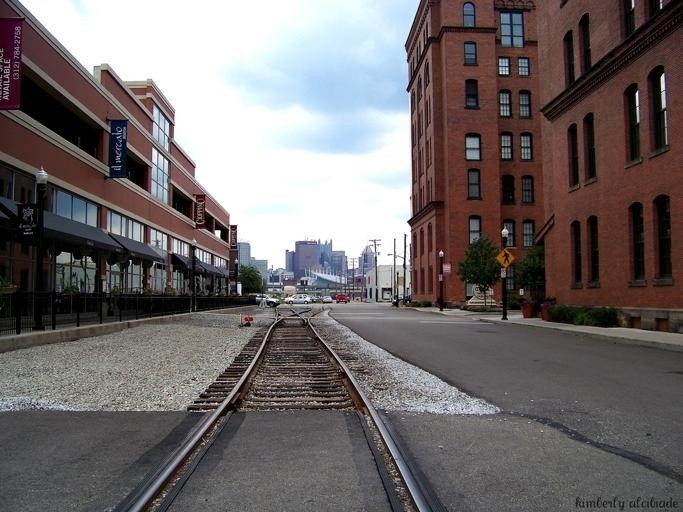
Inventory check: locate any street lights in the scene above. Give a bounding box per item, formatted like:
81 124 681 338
387 254 406 305
234 258 238 293
32 166 48 330
191 238 197 312
501 226 509 320
438 249 444 311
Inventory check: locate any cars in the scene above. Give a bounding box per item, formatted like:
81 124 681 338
336 294 348 303
249 293 332 307
392 295 411 307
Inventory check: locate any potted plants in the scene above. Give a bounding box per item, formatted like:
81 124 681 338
519 299 538 319
540 297 556 320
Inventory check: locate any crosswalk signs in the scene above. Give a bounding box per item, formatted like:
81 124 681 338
496 248 515 268
